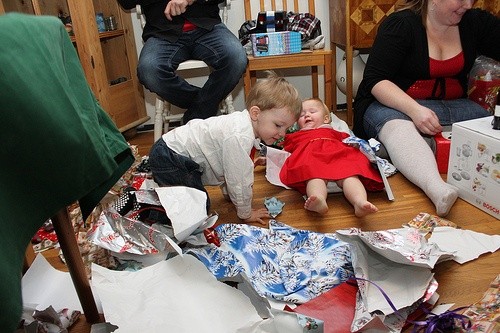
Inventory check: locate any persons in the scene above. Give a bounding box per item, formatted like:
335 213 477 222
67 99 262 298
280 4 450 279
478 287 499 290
117 0 248 127
253 97 384 218
112 75 302 225
352 0 500 218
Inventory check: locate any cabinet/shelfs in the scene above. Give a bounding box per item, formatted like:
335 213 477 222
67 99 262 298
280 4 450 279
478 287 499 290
0 0 151 134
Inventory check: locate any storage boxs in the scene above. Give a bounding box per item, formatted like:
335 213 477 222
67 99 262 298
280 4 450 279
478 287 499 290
251 32 301 57
446 115 500 221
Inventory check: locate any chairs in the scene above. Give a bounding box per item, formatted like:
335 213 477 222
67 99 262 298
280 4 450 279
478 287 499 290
243 0 333 114
136 0 235 143
